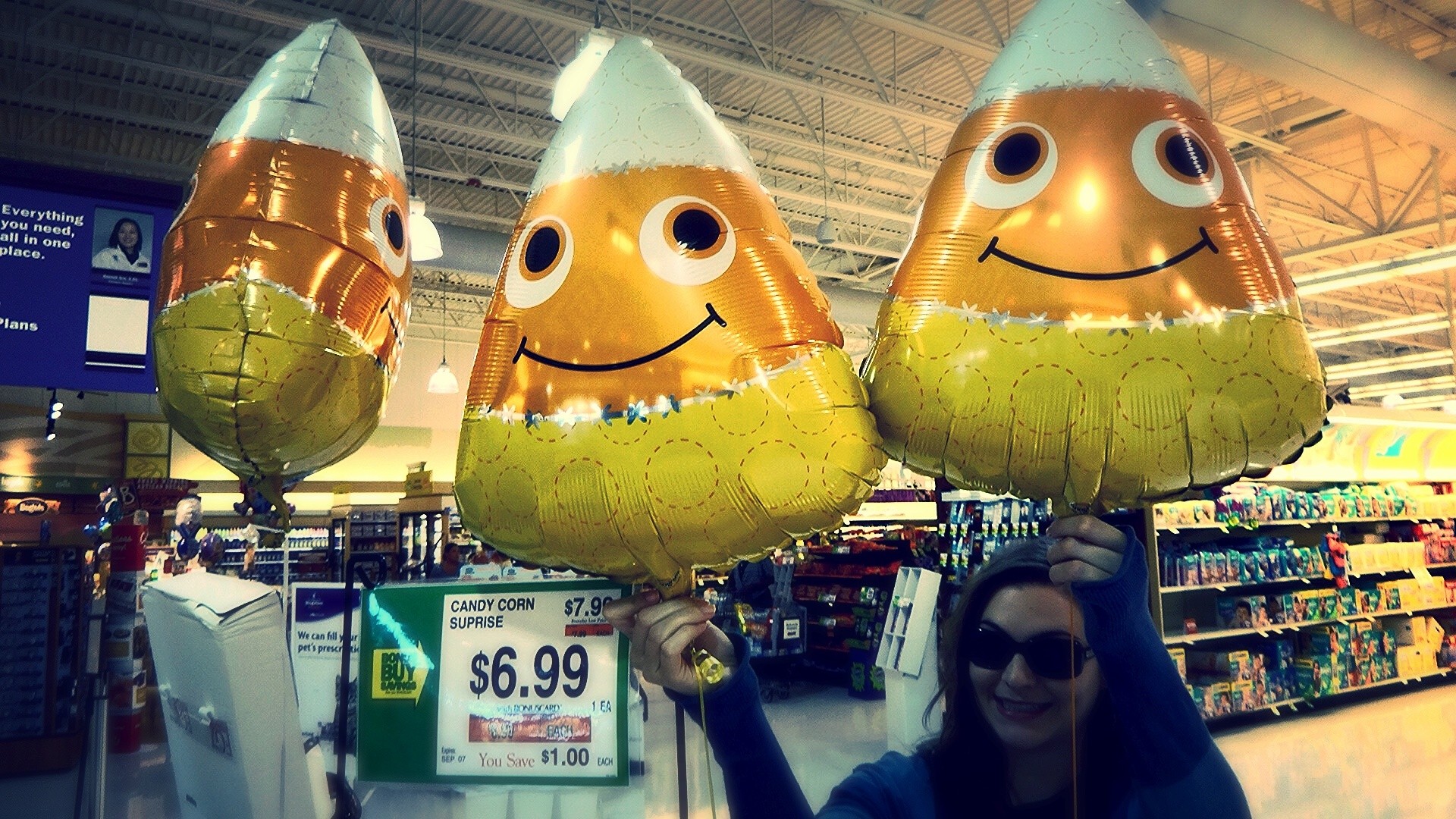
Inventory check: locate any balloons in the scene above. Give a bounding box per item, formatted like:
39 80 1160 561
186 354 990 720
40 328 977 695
149 15 417 530
458 33 888 604
847 0 1336 516
83 497 227 568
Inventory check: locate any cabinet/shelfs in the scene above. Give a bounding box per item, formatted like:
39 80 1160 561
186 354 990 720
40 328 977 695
934 476 1058 605
161 508 334 582
770 520 939 698
329 505 400 583
1142 474 1456 725
397 496 502 581
875 566 945 757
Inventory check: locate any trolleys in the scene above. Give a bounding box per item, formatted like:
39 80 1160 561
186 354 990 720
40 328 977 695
737 605 806 701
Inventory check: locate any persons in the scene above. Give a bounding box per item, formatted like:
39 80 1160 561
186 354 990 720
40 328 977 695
603 508 1255 817
93 217 151 274
1176 498 1397 716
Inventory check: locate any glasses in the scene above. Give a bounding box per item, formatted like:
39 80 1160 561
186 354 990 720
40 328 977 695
963 621 1102 683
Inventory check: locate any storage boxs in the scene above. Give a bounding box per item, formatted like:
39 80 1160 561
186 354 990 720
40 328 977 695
405 469 455 498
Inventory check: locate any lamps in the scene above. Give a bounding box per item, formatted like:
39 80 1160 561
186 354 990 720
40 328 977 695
810 77 839 242
389 0 442 260
423 217 461 393
